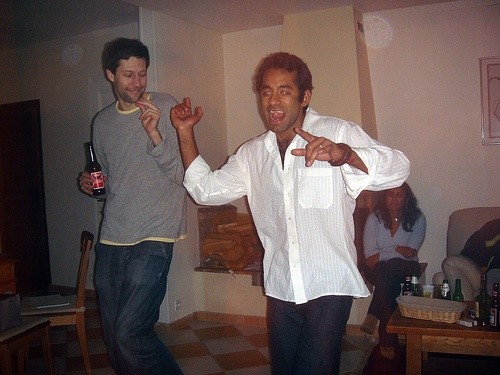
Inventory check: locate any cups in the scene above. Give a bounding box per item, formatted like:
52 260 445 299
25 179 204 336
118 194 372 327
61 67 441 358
422 284 435 298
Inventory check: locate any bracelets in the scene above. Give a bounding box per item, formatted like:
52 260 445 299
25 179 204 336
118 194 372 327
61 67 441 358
329 143 351 166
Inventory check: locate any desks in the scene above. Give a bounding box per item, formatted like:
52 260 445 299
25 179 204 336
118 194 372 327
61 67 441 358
386 301 500 375
0 317 53 375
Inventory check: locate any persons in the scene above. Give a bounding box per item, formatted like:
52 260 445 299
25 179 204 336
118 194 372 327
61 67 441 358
170 52 410 375
362 183 426 360
432 219 500 301
79 38 189 375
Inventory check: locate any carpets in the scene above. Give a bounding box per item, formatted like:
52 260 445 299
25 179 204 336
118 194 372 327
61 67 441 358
362 342 500 375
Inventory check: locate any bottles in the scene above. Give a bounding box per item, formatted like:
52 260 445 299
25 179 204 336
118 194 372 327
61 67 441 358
438 279 451 300
411 272 419 296
488 282 500 331
453 278 464 302
83 142 106 196
474 273 492 326
402 271 414 296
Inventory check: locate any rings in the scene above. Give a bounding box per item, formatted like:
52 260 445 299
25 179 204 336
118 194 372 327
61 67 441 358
319 145 324 149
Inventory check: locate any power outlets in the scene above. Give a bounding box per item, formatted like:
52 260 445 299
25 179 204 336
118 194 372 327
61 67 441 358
176 299 181 310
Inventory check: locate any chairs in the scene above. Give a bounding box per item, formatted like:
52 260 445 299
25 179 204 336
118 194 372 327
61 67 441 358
21 231 94 375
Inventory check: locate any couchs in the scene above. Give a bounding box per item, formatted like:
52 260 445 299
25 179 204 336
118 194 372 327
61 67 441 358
433 207 500 301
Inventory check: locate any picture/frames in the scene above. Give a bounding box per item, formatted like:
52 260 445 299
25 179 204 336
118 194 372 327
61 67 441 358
479 56 500 145
